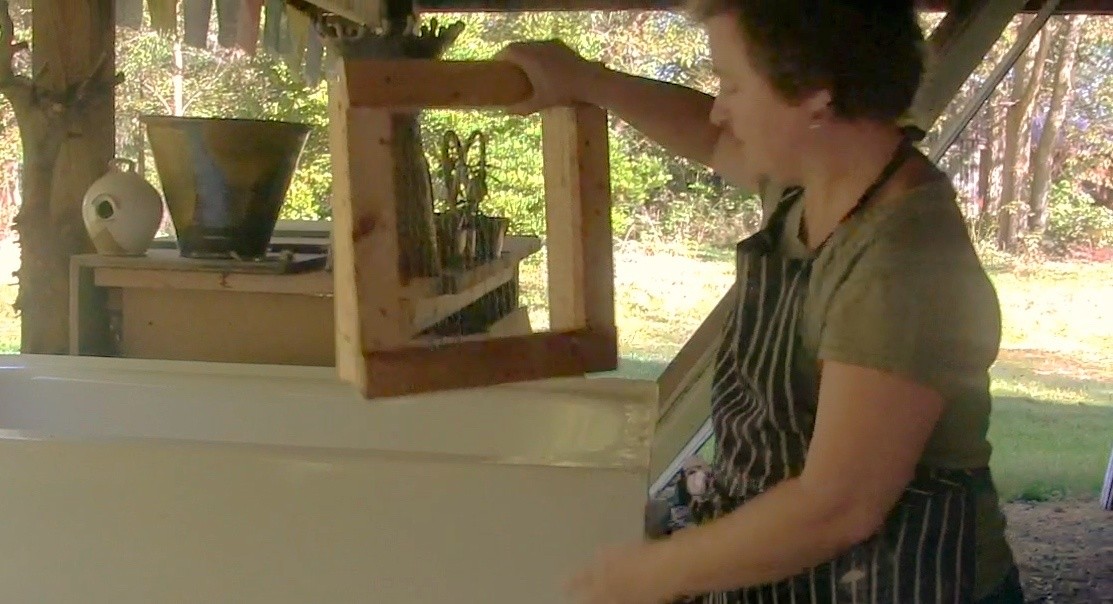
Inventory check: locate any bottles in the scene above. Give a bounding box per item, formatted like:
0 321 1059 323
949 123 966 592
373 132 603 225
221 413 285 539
79 158 163 256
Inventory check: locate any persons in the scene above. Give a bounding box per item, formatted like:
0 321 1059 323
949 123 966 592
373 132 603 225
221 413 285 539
491 0 1024 604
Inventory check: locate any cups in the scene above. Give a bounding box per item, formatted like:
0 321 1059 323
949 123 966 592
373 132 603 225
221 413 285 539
137 113 314 260
